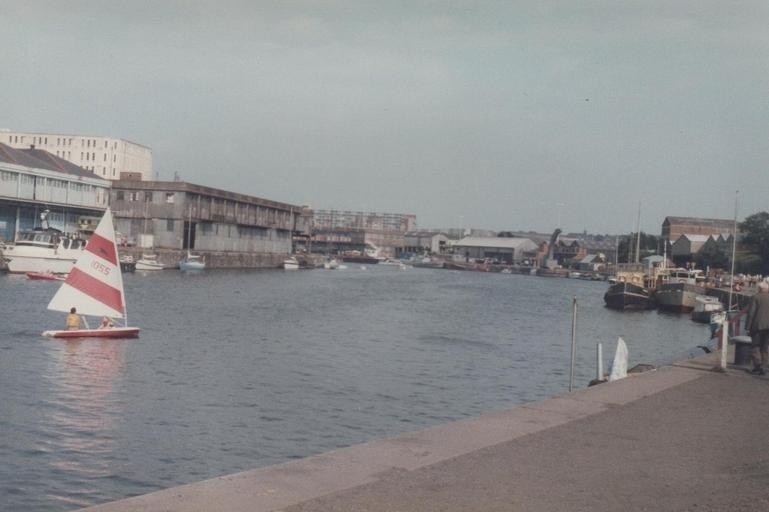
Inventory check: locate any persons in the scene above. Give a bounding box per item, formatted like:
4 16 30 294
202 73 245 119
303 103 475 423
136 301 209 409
64 307 80 331
642 274 655 288
97 316 116 330
744 274 769 375
40 209 50 232
733 282 742 292
712 272 723 288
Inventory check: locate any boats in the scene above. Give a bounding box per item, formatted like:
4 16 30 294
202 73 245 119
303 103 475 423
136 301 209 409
1 209 164 274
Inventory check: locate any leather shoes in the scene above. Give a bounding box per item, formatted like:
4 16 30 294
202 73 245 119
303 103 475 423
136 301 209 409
751 366 766 375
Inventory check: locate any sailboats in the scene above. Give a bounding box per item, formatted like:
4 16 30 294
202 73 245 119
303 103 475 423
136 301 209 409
40 205 140 339
179 208 206 272
603 189 743 338
